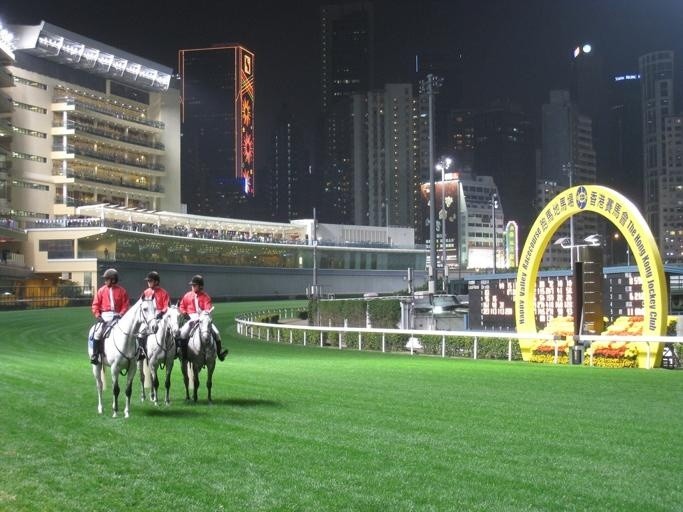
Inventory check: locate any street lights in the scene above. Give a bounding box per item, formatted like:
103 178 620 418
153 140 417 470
544 178 561 271
435 154 453 294
609 233 618 266
417 69 448 295
550 232 601 262
561 159 577 270
488 190 500 276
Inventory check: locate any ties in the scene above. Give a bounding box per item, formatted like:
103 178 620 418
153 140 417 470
110 286 116 311
194 293 203 313
152 289 158 310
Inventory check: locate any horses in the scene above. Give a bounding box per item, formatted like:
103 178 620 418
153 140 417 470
138 298 181 407
181 305 218 405
87 292 159 419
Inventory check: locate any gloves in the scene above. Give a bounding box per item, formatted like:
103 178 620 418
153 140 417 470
97 315 104 324
157 310 165 319
113 313 122 321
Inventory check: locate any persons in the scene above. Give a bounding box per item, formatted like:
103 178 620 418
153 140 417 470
90 268 229 365
104 248 108 261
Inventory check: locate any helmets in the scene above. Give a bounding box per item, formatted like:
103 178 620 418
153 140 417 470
100 268 119 280
188 274 205 285
144 271 160 281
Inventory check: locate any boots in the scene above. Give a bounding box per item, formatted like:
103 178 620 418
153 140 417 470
174 335 189 360
90 340 101 365
216 340 229 361
136 335 147 361
